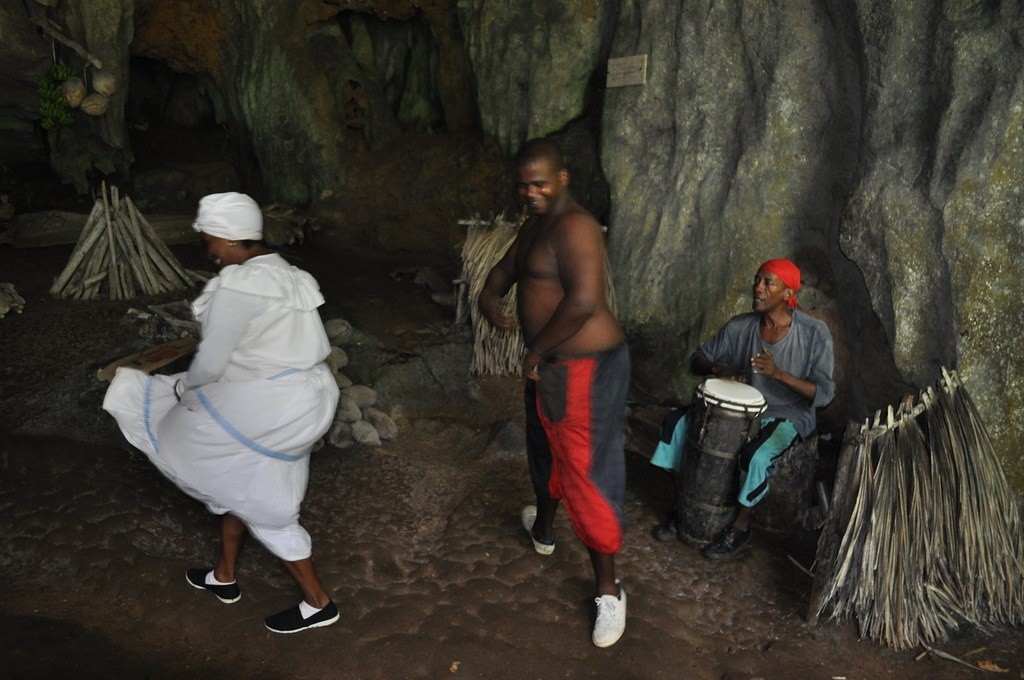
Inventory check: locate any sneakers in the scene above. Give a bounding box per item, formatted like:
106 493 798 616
592 578 627 648
521 505 555 555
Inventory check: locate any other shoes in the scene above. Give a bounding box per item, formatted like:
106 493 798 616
264 598 340 634
185 568 241 604
652 505 678 542
703 517 754 559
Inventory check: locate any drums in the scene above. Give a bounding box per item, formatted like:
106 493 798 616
678 377 767 549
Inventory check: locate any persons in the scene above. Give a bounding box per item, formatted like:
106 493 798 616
649 258 836 563
101 191 340 633
478 136 627 649
